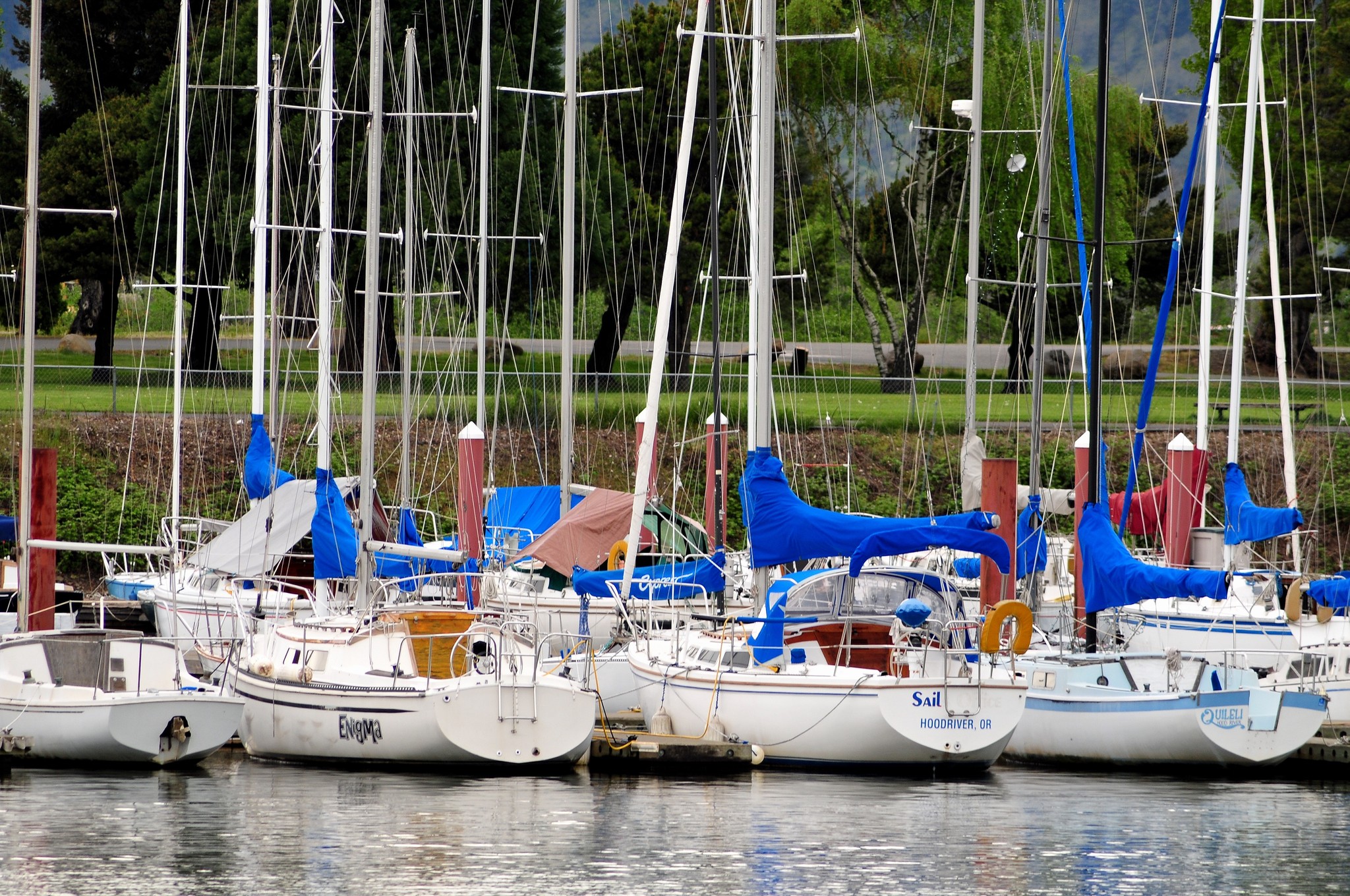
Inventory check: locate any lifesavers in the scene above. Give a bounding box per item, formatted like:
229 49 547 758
607 539 629 572
1284 574 1334 625
980 599 1034 655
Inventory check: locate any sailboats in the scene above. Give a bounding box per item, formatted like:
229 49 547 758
0 0 1350 783
